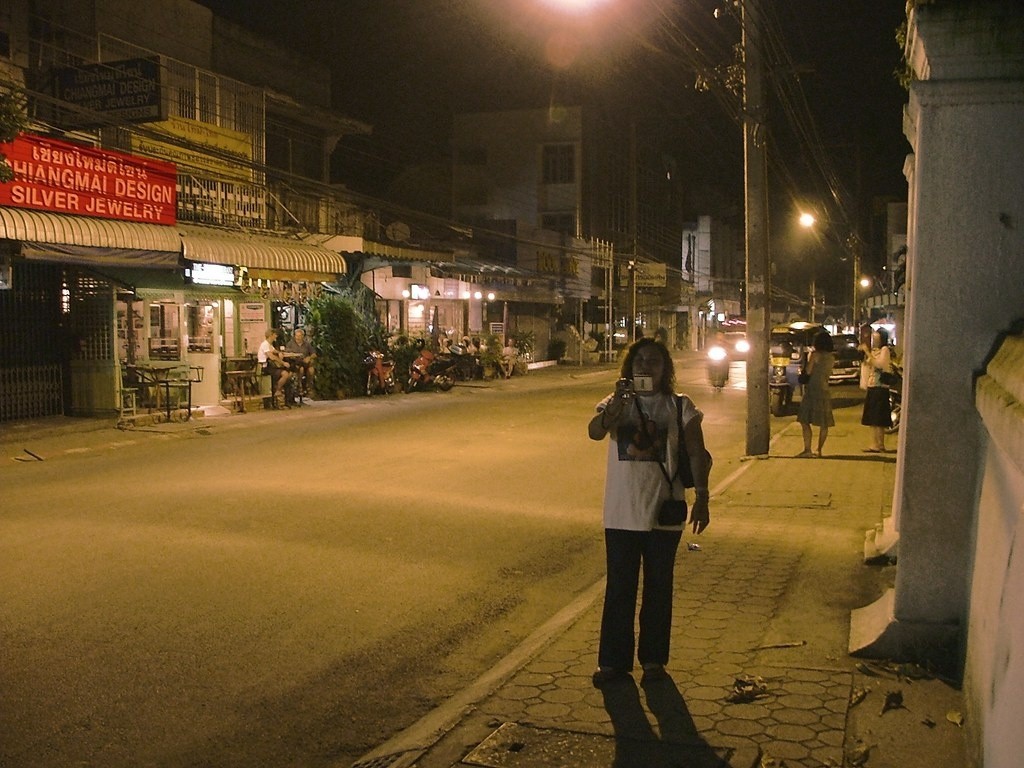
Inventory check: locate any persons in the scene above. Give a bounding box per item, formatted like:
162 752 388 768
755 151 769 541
709 330 730 376
857 328 893 453
588 337 712 682
796 332 835 458
412 330 518 379
635 323 644 342
258 329 316 402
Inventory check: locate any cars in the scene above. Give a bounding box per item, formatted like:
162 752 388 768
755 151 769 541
718 330 747 361
825 333 862 385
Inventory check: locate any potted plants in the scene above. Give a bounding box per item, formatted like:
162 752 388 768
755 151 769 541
476 335 503 379
510 326 538 375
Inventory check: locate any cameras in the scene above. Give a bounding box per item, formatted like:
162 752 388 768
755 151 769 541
619 374 655 392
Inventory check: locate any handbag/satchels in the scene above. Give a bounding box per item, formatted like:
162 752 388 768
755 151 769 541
678 395 713 489
659 501 687 526
879 373 898 386
798 352 808 384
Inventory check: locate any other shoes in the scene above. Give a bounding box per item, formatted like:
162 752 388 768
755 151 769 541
506 373 511 379
796 449 813 459
811 450 821 458
641 661 663 680
600 664 626 681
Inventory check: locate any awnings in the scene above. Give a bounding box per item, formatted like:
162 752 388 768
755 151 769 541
0 206 347 282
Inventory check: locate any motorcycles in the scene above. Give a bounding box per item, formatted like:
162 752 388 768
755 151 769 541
769 326 807 418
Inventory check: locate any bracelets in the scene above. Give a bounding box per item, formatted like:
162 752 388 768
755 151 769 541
695 490 709 497
605 397 622 417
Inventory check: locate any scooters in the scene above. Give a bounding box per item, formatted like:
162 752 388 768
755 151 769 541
704 345 732 394
364 347 396 397
404 338 469 396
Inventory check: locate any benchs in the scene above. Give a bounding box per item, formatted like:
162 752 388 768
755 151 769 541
119 364 139 417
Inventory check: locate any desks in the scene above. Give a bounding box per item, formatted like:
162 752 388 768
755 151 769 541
123 366 177 411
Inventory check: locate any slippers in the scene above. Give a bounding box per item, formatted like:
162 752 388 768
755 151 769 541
861 445 886 453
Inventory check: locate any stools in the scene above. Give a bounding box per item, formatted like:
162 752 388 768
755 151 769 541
227 370 254 413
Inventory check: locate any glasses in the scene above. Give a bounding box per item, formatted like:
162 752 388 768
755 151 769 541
630 357 666 367
